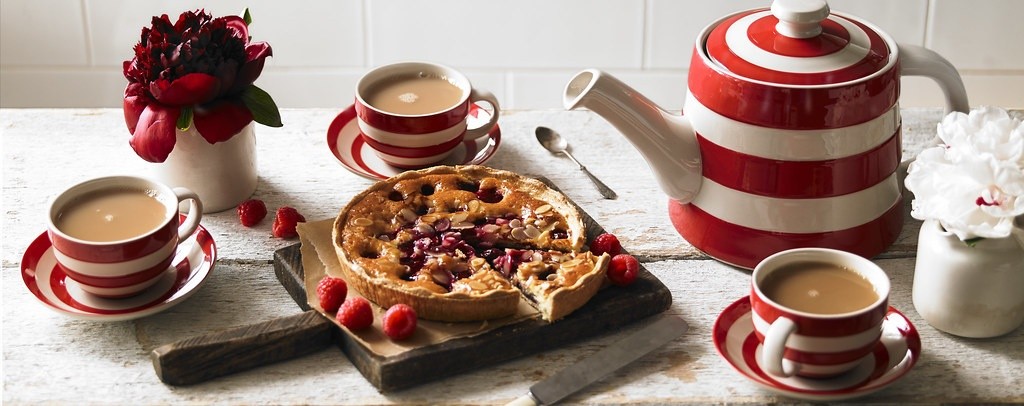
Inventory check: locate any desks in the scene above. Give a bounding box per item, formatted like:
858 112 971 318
0 107 1024 405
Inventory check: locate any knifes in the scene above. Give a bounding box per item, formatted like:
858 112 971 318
509 314 689 406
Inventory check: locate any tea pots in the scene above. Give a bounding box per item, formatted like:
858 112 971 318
562 0 970 271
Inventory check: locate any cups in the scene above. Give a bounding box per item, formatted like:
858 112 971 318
47 174 203 301
750 246 892 380
354 62 500 170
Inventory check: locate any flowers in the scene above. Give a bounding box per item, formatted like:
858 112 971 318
123 9 283 161
904 105 1024 242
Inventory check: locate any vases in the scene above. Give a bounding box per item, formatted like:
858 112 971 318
148 119 258 214
911 219 1024 339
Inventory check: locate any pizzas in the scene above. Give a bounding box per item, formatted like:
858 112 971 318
332 165 610 322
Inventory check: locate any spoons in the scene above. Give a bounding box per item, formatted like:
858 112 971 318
535 126 616 199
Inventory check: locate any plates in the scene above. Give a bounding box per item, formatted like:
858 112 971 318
326 103 501 182
20 213 218 322
711 295 921 400
